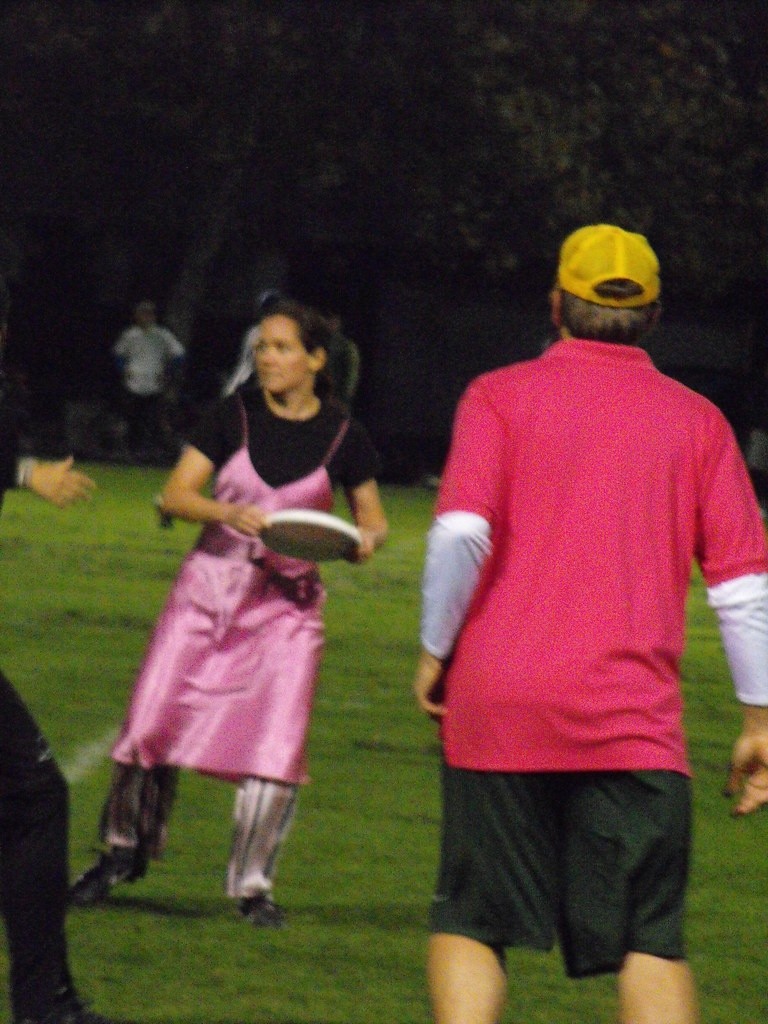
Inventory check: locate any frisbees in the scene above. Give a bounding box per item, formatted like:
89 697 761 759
259 509 363 567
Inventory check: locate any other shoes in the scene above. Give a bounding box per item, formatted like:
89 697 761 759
242 896 281 927
70 856 147 907
12 990 107 1024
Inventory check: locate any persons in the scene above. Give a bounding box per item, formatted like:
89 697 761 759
113 304 188 468
69 299 391 927
0 446 118 1024
221 296 385 480
413 224 767 1024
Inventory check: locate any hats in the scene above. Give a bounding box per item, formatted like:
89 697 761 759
557 223 661 307
